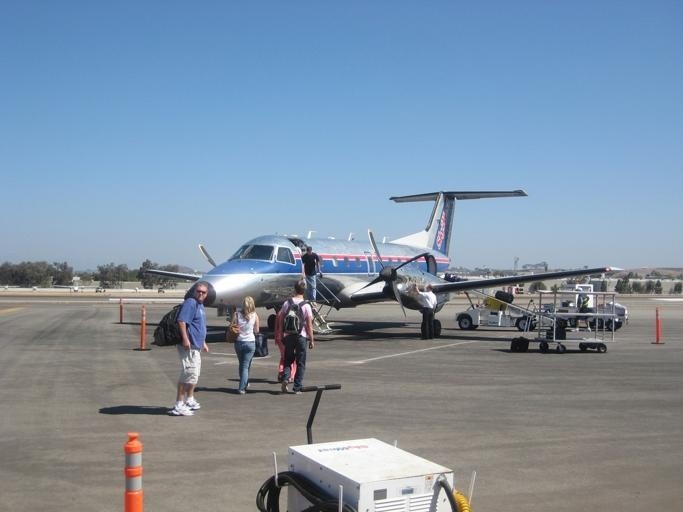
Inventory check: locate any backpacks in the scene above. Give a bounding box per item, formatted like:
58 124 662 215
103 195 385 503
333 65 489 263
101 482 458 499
154 305 183 346
284 296 312 334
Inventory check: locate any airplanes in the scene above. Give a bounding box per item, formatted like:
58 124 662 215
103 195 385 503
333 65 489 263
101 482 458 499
142 188 626 343
0 284 98 293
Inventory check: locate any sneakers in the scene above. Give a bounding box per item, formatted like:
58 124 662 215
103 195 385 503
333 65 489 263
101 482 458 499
282 379 288 391
172 400 200 415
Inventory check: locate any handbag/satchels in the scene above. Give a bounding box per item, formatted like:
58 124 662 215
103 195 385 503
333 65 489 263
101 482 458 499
254 334 268 357
227 313 240 342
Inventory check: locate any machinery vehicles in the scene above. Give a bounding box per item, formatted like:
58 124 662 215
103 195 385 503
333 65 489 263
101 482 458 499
541 273 629 333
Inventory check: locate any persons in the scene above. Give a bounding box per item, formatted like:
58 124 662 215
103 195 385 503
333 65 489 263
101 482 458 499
273 308 295 384
276 279 314 394
169 281 210 415
300 246 324 307
569 287 592 332
228 295 260 394
413 283 438 338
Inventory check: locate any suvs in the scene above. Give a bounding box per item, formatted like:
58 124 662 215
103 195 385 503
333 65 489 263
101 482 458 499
95 287 105 293
157 286 164 293
456 291 537 332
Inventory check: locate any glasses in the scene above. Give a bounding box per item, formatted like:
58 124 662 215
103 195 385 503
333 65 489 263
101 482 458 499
196 290 206 293
